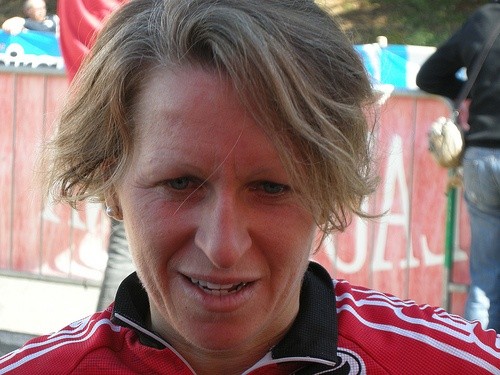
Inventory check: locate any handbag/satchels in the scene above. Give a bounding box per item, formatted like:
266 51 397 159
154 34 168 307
428 120 464 165
